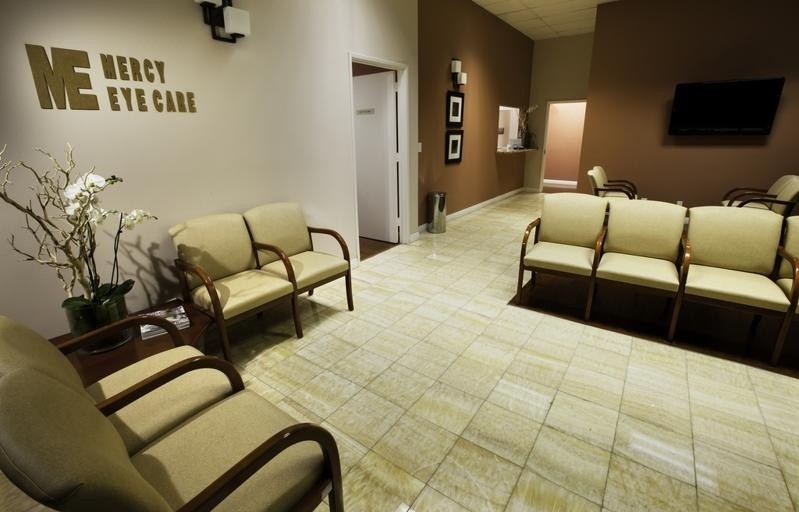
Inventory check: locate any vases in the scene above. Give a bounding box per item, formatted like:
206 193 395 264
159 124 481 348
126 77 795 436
61 284 133 355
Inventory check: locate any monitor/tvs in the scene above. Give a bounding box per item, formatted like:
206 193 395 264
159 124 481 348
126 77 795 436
668 76 785 136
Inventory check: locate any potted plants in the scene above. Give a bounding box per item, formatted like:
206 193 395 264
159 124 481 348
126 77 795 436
515 104 540 148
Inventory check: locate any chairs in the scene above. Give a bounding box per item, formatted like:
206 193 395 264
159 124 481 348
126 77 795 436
166 201 354 362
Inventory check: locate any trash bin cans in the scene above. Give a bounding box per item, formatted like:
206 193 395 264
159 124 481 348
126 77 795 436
426 191 447 234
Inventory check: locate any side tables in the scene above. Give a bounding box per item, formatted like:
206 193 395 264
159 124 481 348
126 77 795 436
48 297 214 388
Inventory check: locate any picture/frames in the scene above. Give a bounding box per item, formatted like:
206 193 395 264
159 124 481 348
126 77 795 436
445 130 463 163
446 90 464 127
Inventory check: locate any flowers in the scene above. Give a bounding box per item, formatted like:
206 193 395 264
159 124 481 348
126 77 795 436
0 142 158 300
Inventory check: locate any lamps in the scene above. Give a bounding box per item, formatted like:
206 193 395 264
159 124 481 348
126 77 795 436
451 58 467 85
196 0 250 43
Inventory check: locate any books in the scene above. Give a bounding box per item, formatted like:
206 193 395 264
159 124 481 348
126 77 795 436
137 306 190 341
